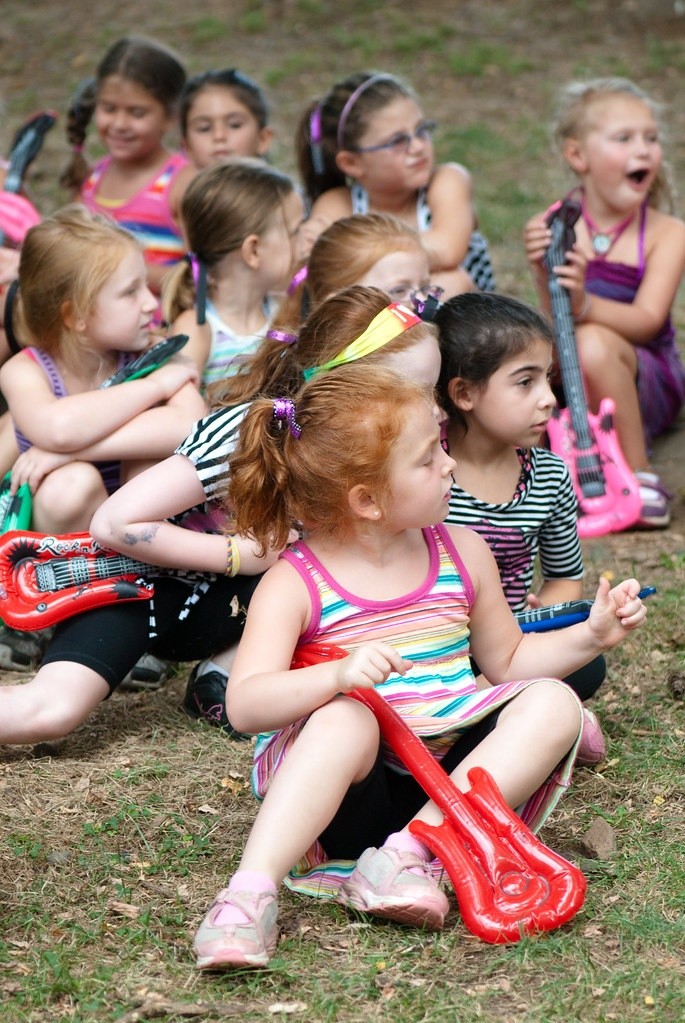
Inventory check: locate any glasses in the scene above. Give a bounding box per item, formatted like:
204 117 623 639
387 283 444 307
355 121 437 155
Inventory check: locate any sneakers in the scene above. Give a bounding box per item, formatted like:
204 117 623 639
194 886 277 969
183 660 254 738
131 651 170 688
334 847 450 930
0 621 53 671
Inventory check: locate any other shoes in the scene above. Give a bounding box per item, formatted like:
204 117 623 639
577 707 606 765
633 470 675 528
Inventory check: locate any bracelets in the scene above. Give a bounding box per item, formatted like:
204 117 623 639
574 295 591 321
225 535 240 576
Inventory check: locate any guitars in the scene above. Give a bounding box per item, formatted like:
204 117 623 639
288 631 587 946
0 531 166 632
543 189 648 538
0 334 191 531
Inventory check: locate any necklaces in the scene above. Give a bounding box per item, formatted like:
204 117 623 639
582 195 637 254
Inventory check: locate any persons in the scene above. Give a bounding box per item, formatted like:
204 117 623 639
57 36 201 334
171 156 308 392
425 293 651 764
0 202 208 688
276 213 430 326
524 78 684 528
296 70 495 305
194 362 584 977
179 69 273 169
0 280 441 743
